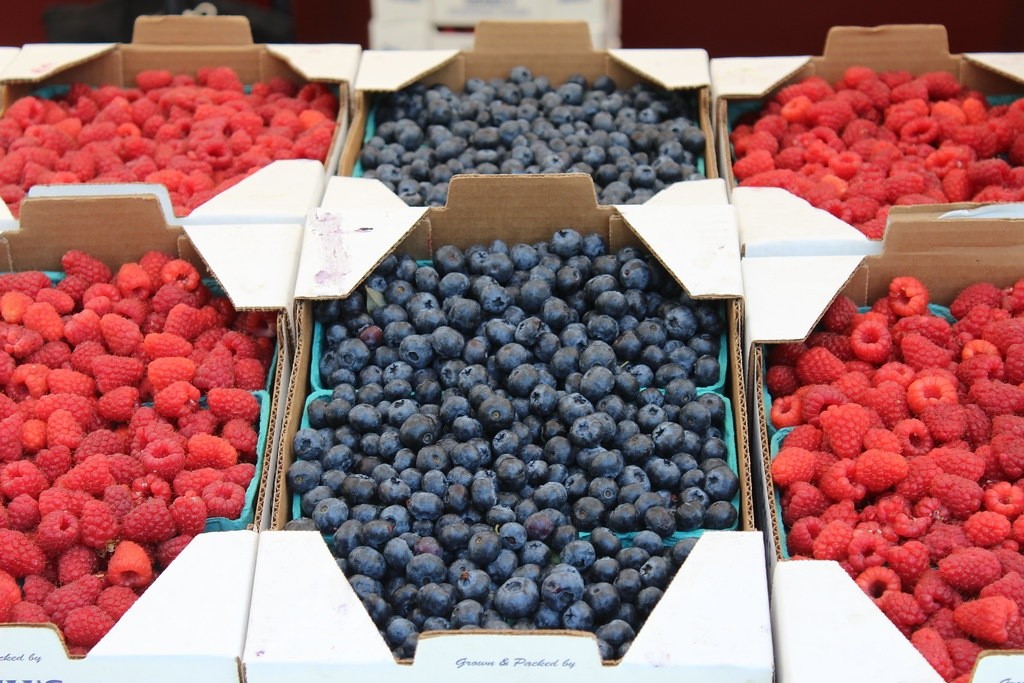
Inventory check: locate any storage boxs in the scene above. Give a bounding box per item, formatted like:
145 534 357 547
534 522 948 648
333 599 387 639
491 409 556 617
0 16 1024 683
